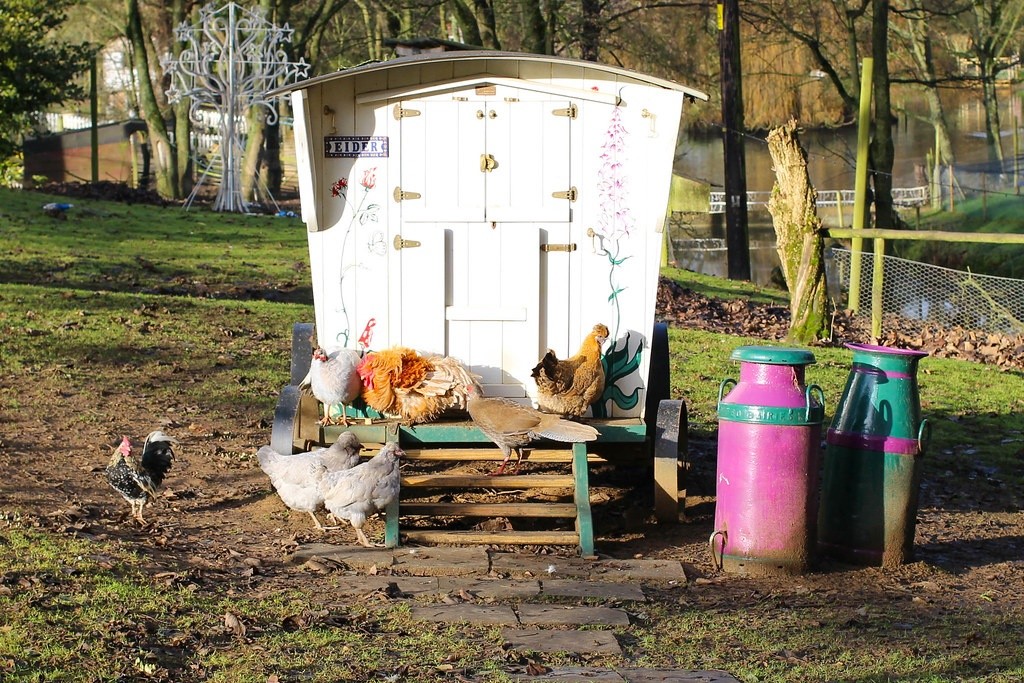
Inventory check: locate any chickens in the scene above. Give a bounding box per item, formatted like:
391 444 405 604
257 323 610 548
105 430 179 527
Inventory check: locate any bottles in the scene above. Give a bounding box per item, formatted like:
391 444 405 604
823 343 934 566
706 345 825 574
